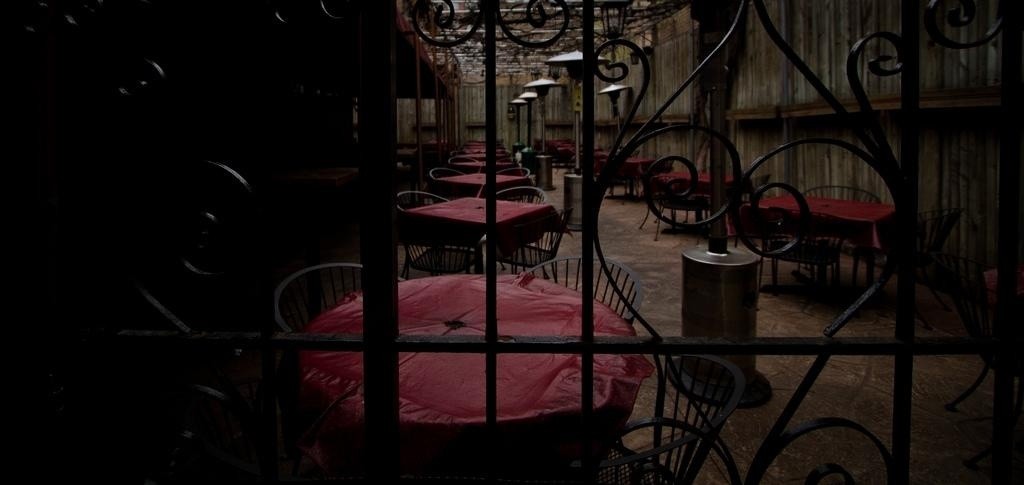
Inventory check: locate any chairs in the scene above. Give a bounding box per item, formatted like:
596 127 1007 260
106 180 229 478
274 140 989 244
914 208 966 330
566 353 745 485
526 254 645 325
771 208 849 298
395 190 478 280
274 262 362 335
496 208 575 281
